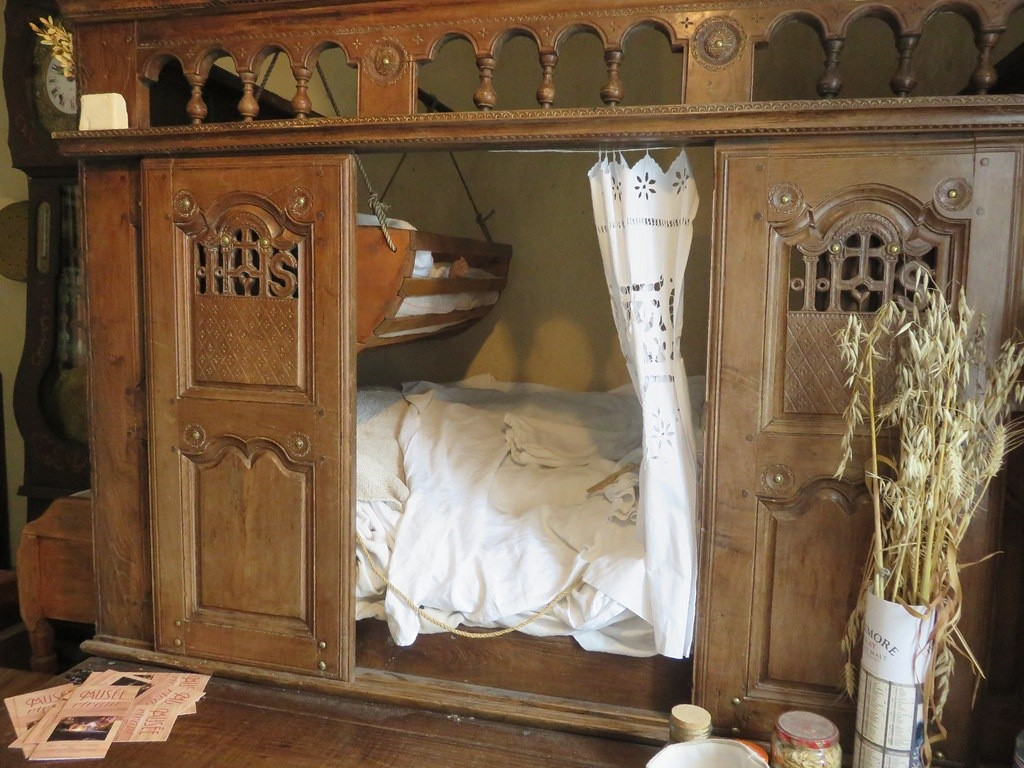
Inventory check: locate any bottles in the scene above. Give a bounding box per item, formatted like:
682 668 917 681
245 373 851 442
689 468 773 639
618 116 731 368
769 710 843 767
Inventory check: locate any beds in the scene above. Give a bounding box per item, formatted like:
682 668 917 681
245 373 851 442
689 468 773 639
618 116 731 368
355 373 708 662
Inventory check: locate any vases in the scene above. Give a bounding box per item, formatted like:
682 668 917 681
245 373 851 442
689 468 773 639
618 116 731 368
853 582 937 768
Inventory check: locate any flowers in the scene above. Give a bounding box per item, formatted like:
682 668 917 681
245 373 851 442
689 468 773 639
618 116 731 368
823 264 1024 602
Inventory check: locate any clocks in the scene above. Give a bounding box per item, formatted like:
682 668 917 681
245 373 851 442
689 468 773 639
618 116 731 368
44 48 77 116
